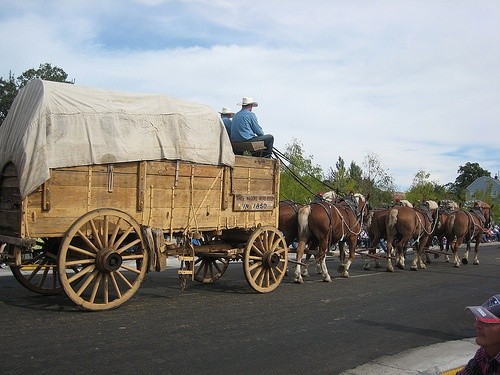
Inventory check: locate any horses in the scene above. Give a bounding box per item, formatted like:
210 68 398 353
370 203 495 272
278 190 371 284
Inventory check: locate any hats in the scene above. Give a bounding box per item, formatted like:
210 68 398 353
465 295 500 323
218 107 235 118
237 97 258 107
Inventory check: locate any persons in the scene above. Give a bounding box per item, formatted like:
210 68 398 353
217 107 236 138
292 224 500 252
455 295 500 375
0 241 9 268
230 98 274 158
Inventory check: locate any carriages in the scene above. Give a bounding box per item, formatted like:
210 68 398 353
0 77 494 311
